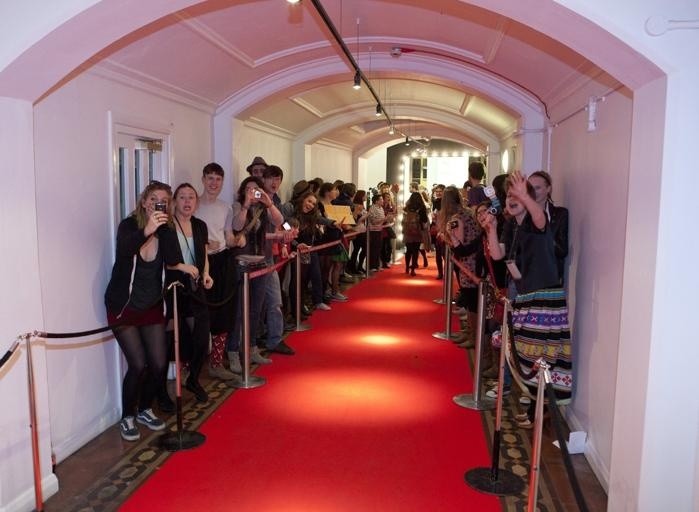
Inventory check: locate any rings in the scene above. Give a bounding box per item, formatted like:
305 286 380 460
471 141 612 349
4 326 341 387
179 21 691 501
156 215 159 217
210 285 213 287
159 218 161 221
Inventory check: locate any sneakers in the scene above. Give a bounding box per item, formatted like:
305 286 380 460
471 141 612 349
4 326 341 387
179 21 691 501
136 409 167 431
181 383 208 402
347 260 402 275
331 292 348 299
156 394 176 413
483 346 513 397
315 303 331 310
208 367 237 380
120 417 141 442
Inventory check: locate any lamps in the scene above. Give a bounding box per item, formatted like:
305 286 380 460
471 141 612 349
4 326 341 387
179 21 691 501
291 0 428 158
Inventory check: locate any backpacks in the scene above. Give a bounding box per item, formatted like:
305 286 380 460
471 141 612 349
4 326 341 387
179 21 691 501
403 209 420 237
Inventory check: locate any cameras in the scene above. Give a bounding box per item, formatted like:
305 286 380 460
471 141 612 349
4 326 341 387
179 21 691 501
253 187 261 199
490 207 503 215
450 221 458 229
155 202 166 213
191 275 204 291
282 221 291 231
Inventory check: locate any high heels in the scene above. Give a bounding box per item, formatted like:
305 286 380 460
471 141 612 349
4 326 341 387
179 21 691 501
516 392 553 430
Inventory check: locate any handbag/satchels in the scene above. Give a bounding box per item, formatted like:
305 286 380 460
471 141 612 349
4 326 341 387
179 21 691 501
493 288 508 325
178 274 205 298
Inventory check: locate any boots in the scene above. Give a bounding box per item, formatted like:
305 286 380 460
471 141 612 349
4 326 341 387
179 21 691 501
227 351 242 372
249 346 271 364
420 249 428 267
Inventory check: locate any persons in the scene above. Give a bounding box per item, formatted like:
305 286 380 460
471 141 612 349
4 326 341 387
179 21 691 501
182 161 247 385
159 182 214 413
103 180 183 441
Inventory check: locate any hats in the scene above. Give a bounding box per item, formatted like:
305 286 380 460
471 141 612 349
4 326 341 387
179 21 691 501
294 180 314 197
246 156 267 171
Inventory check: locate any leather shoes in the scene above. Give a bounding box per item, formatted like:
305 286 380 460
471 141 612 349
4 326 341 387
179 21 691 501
452 306 474 349
276 341 294 354
286 303 312 331
